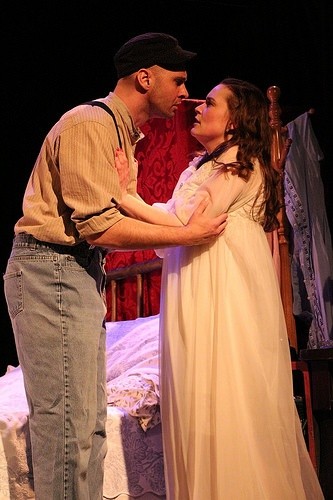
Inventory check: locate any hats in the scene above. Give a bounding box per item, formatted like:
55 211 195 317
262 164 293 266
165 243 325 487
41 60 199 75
113 33 198 78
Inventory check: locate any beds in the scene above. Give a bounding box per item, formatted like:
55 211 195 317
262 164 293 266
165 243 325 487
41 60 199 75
0 258 164 500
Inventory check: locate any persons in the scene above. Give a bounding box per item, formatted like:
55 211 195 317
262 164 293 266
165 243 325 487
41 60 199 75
112 79 327 500
4 32 228 500
